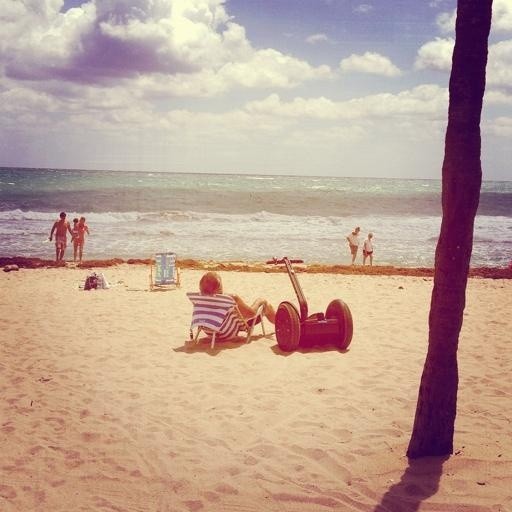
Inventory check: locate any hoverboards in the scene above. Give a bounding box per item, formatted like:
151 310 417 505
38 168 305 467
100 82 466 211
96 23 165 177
262 255 356 352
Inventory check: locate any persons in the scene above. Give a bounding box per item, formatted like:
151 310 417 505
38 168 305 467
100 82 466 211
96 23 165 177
72 217 90 261
50 211 76 262
200 272 277 327
71 218 80 243
347 228 360 264
362 232 373 265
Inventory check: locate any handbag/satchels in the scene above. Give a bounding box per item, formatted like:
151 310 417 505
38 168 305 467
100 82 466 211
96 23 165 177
83 272 110 291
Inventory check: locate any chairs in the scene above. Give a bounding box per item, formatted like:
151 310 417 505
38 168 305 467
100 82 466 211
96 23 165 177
183 290 269 350
150 252 182 290
